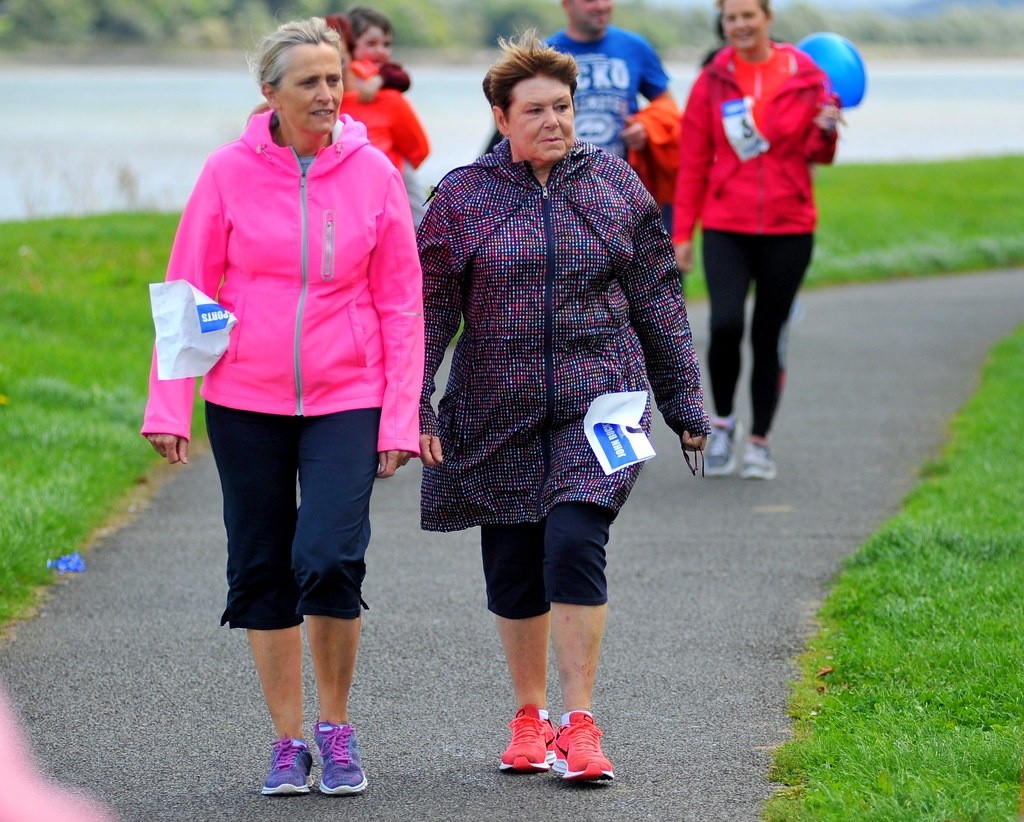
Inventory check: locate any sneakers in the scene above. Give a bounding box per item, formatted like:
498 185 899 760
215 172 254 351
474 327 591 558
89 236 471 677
706 415 778 480
265 718 366 795
500 706 614 781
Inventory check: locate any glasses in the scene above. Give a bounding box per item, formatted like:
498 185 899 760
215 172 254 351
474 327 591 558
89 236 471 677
679 430 706 476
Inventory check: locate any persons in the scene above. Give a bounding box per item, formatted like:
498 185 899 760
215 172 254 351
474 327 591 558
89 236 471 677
343 5 412 104
400 25 711 784
144 18 425 798
671 0 841 480
320 9 431 236
537 0 682 230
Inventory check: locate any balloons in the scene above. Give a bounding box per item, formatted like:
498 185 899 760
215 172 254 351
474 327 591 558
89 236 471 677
795 32 865 106
